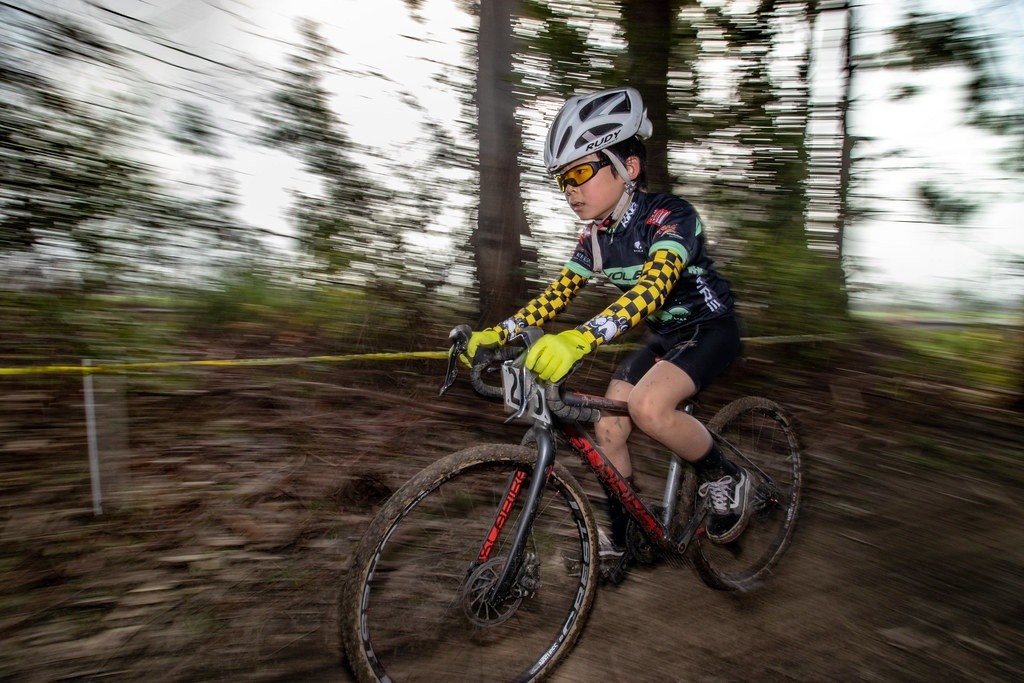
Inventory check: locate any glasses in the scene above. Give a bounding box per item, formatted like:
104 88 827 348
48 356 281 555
553 158 613 192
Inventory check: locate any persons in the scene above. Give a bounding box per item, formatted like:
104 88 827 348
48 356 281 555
448 87 752 562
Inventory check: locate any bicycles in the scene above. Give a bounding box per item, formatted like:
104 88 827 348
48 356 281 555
336 325 808 682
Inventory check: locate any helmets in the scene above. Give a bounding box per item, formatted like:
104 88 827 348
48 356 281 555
543 87 654 175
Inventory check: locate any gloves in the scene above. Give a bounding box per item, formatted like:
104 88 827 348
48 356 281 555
526 326 603 383
446 324 507 372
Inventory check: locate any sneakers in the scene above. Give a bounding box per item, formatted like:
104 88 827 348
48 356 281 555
698 460 752 545
562 526 627 561
